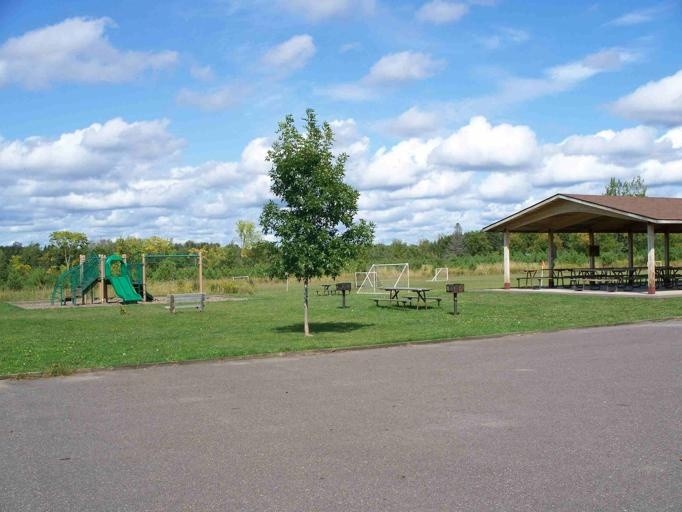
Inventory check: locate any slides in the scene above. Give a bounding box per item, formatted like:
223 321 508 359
105 255 142 303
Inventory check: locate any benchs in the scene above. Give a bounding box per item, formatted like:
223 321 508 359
164 293 206 314
516 265 682 292
402 295 442 306
313 289 336 295
367 297 409 311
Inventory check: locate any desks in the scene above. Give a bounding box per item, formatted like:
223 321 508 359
319 284 332 295
384 286 431 311
